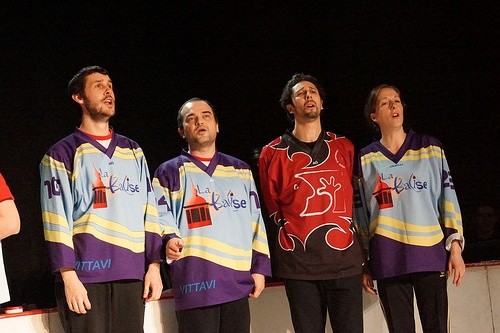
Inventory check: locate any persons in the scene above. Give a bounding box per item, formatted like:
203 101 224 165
258 73 364 333
39 65 163 333
0 172 21 305
352 83 466 333
152 97 272 333
